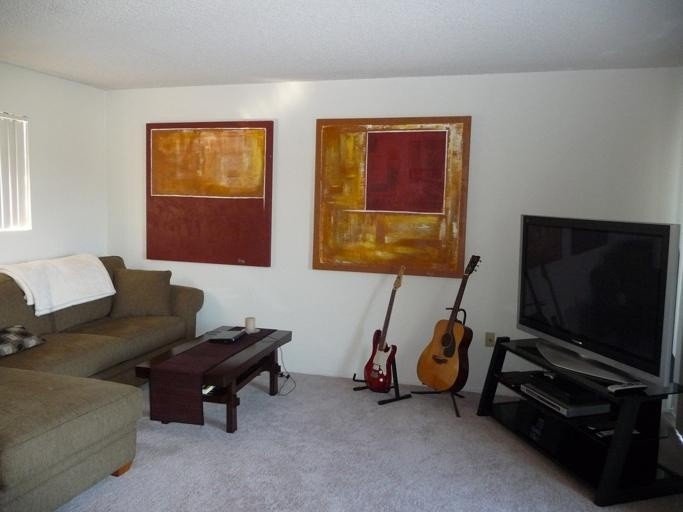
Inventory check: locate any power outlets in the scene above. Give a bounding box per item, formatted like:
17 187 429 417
484 333 496 347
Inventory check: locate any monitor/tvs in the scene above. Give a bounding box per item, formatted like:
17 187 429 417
515 213 681 390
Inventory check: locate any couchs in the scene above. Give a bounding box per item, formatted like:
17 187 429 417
0 256 205 512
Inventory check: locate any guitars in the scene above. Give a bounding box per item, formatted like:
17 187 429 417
417 255 482 393
364 265 407 393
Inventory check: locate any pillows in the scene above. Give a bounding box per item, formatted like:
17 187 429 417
110 268 173 319
0 324 44 358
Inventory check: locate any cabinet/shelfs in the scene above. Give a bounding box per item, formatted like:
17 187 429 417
476 336 683 507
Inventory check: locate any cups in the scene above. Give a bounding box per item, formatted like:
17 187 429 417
245 317 255 331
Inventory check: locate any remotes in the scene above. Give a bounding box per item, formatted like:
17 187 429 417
607 381 647 394
